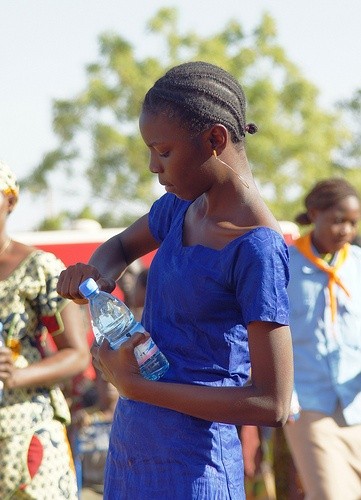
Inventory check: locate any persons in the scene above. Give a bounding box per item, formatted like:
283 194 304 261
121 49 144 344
57 61 294 500
67 258 151 500
280 175 361 499
1 167 92 500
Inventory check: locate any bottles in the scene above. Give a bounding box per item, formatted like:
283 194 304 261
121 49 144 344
78 277 171 381
